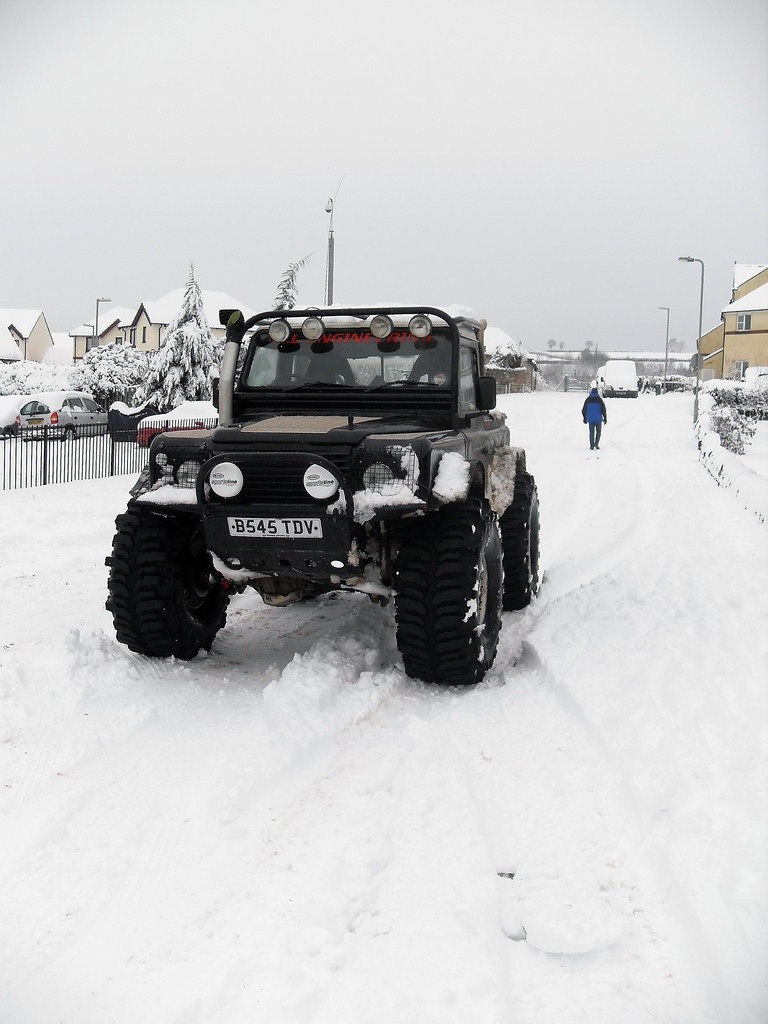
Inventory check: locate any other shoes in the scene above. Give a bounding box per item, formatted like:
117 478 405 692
594 443 599 449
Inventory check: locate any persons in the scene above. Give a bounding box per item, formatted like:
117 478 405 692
582 388 607 450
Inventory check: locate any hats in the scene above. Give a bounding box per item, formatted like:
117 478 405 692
591 388 598 394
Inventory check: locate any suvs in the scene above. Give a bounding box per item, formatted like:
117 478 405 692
106 305 539 687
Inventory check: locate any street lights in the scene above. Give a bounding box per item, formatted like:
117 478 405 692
325 196 335 305
678 256 704 424
659 306 670 394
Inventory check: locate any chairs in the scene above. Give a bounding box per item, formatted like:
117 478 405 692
304 354 359 387
404 348 452 387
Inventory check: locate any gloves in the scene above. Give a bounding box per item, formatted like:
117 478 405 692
583 418 587 424
603 418 607 425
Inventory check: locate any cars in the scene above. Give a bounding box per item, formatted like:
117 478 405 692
137 400 220 450
0 395 28 440
19 392 108 443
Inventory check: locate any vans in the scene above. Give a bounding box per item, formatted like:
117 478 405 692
587 360 638 398
